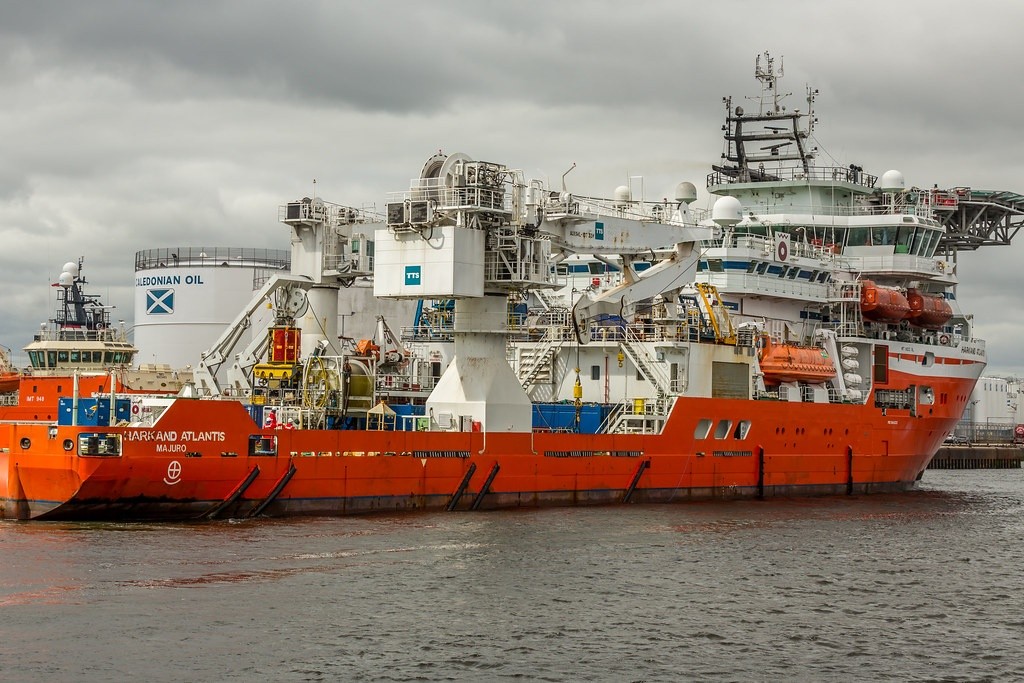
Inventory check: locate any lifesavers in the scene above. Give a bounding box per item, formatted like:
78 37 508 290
262 411 275 429
940 336 948 344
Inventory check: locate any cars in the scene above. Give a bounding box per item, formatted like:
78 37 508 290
945 434 970 443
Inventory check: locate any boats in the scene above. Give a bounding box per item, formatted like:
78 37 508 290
755 335 836 382
846 279 909 323
0 48 1024 519
903 288 954 330
